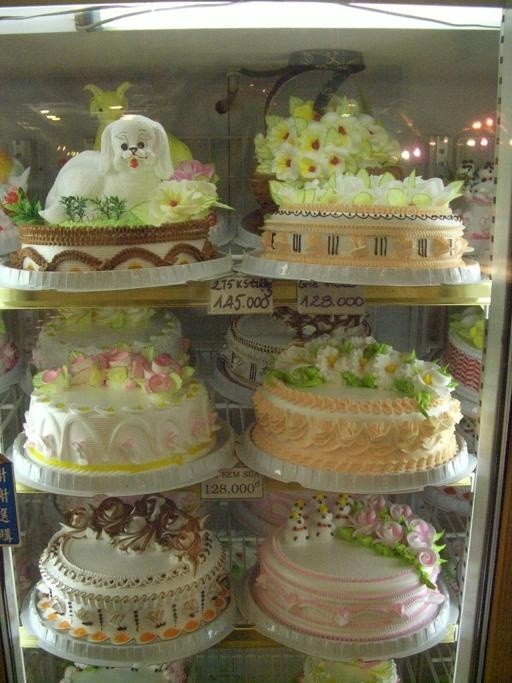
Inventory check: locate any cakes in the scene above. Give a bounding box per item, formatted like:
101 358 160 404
253 491 449 642
0 81 234 272
33 495 234 644
296 654 402 683
218 306 371 394
31 306 193 372
21 343 223 475
245 95 496 269
437 304 487 405
58 660 188 683
236 484 386 537
249 332 463 476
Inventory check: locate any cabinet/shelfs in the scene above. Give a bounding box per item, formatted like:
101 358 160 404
2 3 511 683
